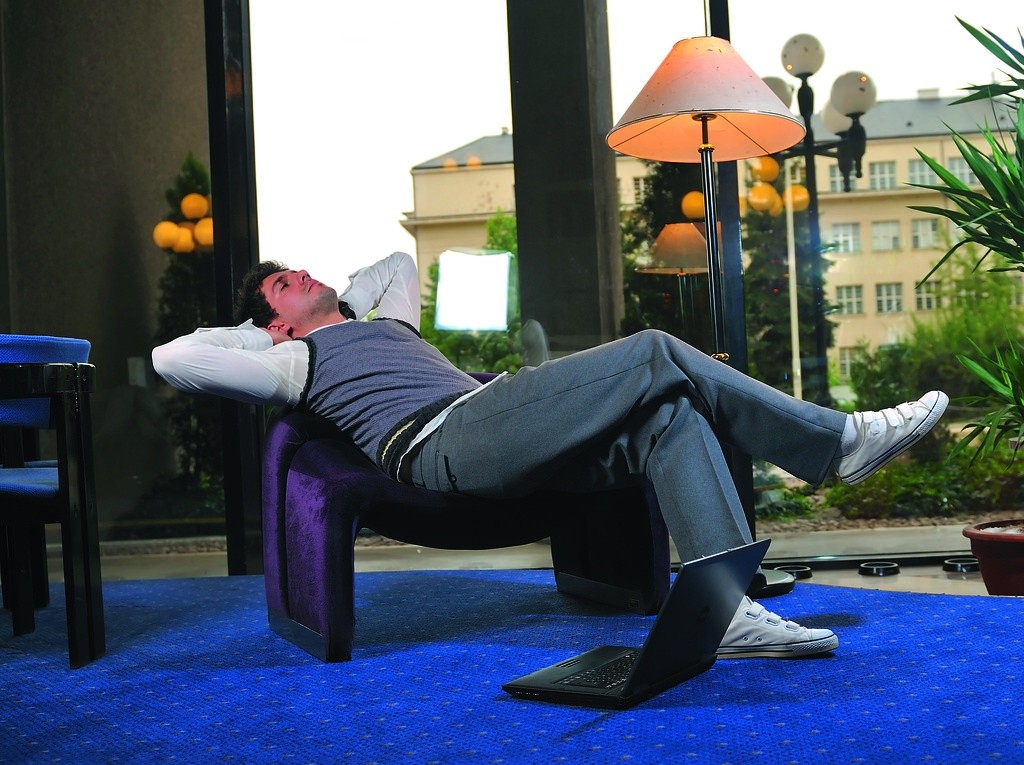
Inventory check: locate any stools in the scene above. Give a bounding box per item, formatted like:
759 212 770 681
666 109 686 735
263 372 672 665
1 460 59 635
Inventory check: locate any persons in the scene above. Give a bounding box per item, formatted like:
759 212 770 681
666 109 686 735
152 251 950 659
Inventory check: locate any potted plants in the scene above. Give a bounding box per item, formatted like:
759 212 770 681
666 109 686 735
905 12 1024 595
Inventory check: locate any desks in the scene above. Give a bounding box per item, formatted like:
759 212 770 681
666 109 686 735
0 333 108 668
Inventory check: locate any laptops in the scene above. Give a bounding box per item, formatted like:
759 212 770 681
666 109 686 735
501 538 773 712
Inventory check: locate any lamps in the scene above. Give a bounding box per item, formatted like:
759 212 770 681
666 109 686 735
635 222 709 351
605 36 806 597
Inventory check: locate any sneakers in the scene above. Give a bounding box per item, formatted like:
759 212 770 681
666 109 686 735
832 389 950 486
715 594 838 659
